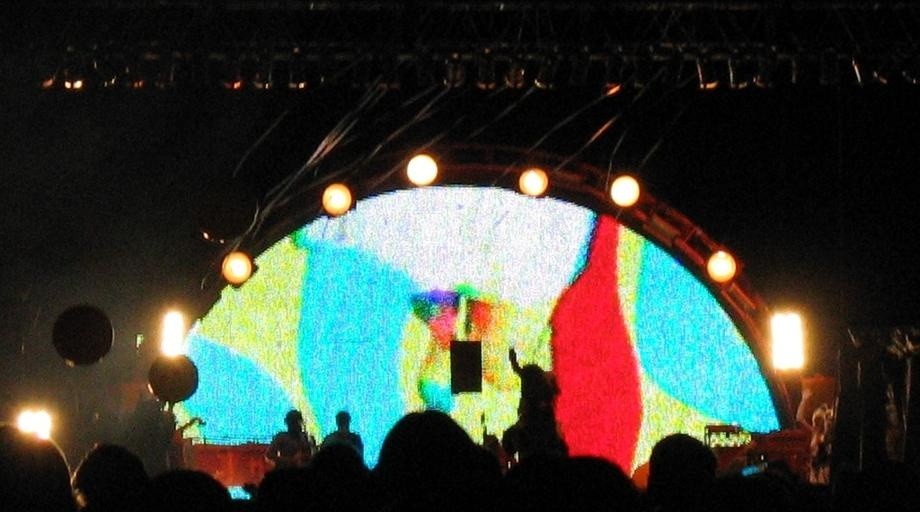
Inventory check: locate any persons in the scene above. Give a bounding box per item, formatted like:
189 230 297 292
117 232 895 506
512 350 557 428
